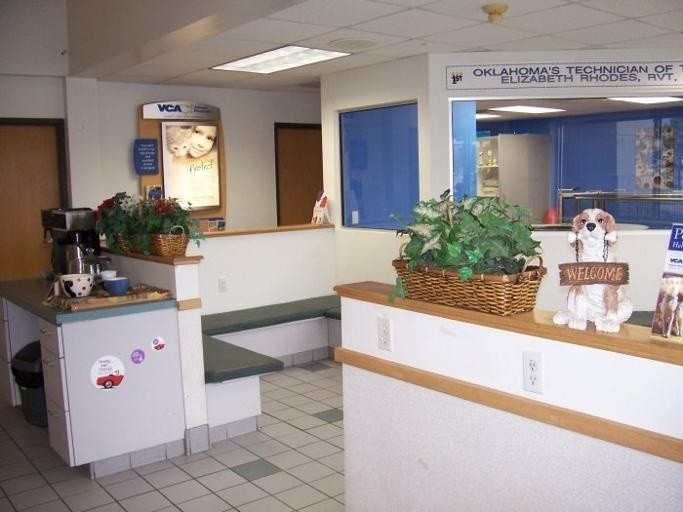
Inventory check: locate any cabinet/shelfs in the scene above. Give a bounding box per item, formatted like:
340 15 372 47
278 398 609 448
476 134 551 223
37 318 76 467
0 298 18 407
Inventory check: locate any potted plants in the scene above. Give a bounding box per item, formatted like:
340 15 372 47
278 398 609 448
388 189 547 314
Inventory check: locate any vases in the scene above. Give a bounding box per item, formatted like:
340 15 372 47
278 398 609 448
134 226 191 256
113 232 141 256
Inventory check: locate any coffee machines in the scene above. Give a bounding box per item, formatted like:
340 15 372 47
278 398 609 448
41 206 112 274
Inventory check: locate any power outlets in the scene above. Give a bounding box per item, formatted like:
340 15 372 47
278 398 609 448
523 353 543 395
377 317 391 351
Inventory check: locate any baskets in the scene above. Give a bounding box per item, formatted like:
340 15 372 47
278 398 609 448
392 242 546 316
109 225 189 256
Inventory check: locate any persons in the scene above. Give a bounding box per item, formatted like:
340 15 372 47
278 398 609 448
187 126 217 159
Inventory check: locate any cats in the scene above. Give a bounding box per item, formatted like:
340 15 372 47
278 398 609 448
166 124 198 158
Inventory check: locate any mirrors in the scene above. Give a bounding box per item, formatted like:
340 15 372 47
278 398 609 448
448 92 683 235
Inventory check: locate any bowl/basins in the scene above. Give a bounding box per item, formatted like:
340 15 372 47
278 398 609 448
103 277 129 296
99 270 118 279
59 273 96 297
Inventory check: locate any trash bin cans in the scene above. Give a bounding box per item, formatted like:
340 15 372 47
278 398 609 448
10 340 48 428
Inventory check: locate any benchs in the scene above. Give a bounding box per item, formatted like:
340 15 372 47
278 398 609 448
200 294 341 442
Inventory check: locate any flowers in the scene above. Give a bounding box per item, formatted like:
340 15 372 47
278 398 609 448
134 197 205 256
96 191 138 254
